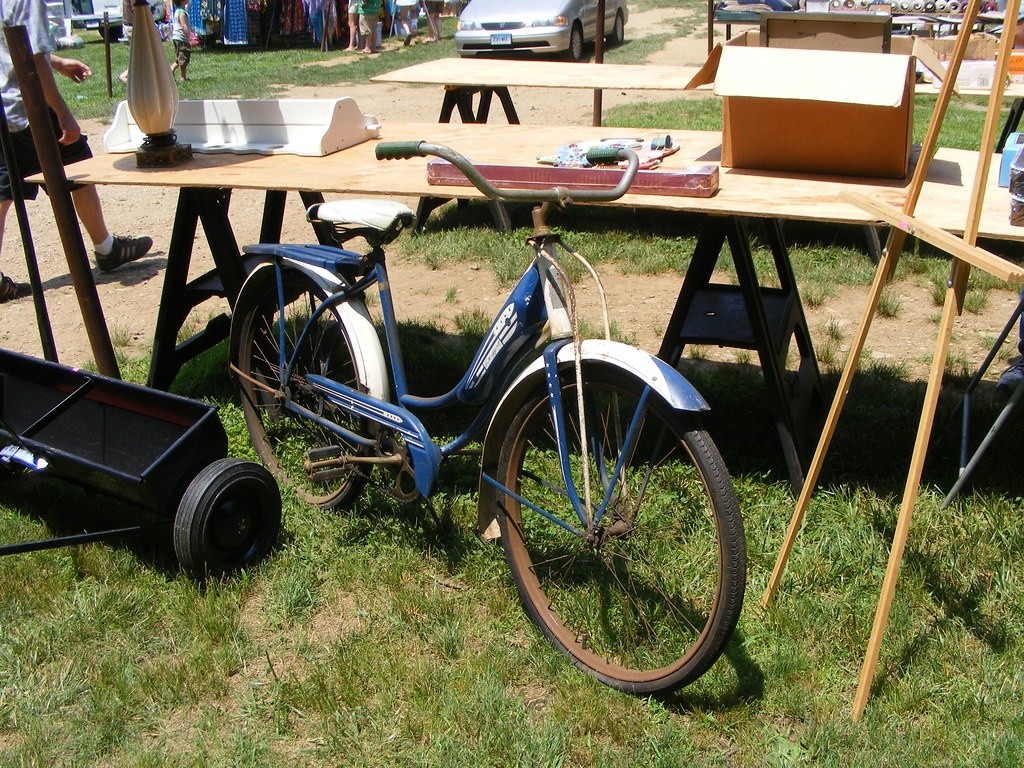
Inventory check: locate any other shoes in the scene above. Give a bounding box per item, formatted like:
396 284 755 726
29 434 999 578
404 33 413 46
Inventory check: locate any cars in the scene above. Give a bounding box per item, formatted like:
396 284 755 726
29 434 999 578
454 0 629 61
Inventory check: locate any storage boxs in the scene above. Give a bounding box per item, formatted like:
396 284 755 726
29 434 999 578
998 132 1024 187
684 29 960 181
923 31 996 90
868 4 892 15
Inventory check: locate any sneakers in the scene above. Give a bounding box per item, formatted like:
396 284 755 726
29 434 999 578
95 233 153 273
996 358 1024 388
0 272 32 304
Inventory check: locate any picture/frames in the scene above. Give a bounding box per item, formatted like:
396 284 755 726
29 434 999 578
760 10 892 55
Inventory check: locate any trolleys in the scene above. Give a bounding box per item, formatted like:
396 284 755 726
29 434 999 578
0 348 283 589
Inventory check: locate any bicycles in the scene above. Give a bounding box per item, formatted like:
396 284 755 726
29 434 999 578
227 142 746 693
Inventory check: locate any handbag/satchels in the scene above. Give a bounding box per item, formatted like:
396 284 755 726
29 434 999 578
188 31 200 46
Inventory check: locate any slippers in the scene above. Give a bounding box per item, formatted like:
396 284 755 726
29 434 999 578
117 75 128 86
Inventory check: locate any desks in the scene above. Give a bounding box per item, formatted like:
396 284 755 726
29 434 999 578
369 58 713 127
25 121 1024 497
893 15 985 35
715 8 769 23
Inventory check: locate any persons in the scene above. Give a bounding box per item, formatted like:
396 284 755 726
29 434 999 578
396 0 444 47
169 0 193 81
119 0 137 85
343 0 382 54
0 0 153 301
